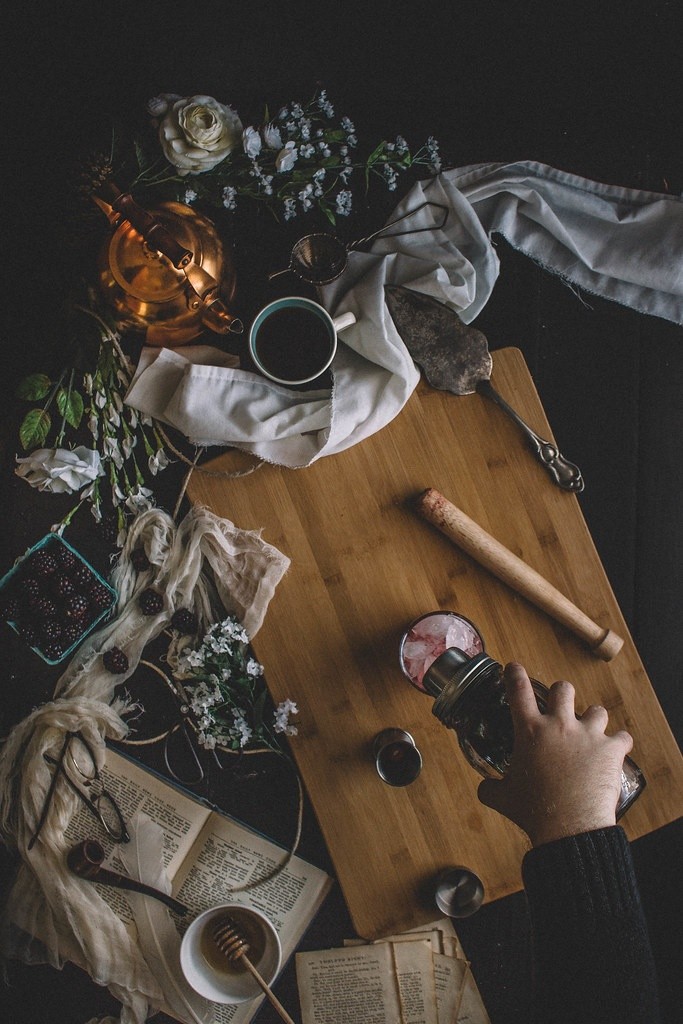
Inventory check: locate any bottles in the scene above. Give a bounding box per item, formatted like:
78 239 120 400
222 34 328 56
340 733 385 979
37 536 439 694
422 646 647 824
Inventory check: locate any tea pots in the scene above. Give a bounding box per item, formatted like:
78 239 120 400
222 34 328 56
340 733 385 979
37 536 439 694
91 179 247 349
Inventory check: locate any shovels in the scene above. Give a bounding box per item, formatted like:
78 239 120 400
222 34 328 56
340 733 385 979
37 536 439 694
383 281 586 495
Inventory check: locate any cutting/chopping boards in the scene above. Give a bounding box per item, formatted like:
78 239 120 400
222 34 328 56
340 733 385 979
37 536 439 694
182 345 683 940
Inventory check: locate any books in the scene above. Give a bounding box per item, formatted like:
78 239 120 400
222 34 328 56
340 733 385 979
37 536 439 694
8 735 333 1024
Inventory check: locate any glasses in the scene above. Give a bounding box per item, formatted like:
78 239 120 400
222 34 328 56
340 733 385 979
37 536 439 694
27 731 131 849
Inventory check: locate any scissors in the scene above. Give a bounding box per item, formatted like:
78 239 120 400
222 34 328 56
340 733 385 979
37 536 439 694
164 677 244 785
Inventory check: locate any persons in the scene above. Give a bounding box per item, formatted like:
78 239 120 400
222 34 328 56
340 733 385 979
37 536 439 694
475 662 683 1024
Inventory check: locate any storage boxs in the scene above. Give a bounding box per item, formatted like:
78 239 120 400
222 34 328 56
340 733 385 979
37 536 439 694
0 532 118 665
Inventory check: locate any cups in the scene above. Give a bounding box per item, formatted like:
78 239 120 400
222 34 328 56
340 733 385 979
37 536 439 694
248 296 356 386
398 610 486 698
434 863 485 920
372 728 423 787
180 903 282 1005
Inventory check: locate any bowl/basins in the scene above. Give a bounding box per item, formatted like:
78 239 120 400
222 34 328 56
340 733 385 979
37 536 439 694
0 532 119 665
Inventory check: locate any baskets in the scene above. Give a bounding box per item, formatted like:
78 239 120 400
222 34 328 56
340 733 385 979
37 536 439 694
0 532 118 665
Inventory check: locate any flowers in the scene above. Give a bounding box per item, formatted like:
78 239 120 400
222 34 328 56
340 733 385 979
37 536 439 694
129 86 444 224
12 314 176 551
164 620 301 774
